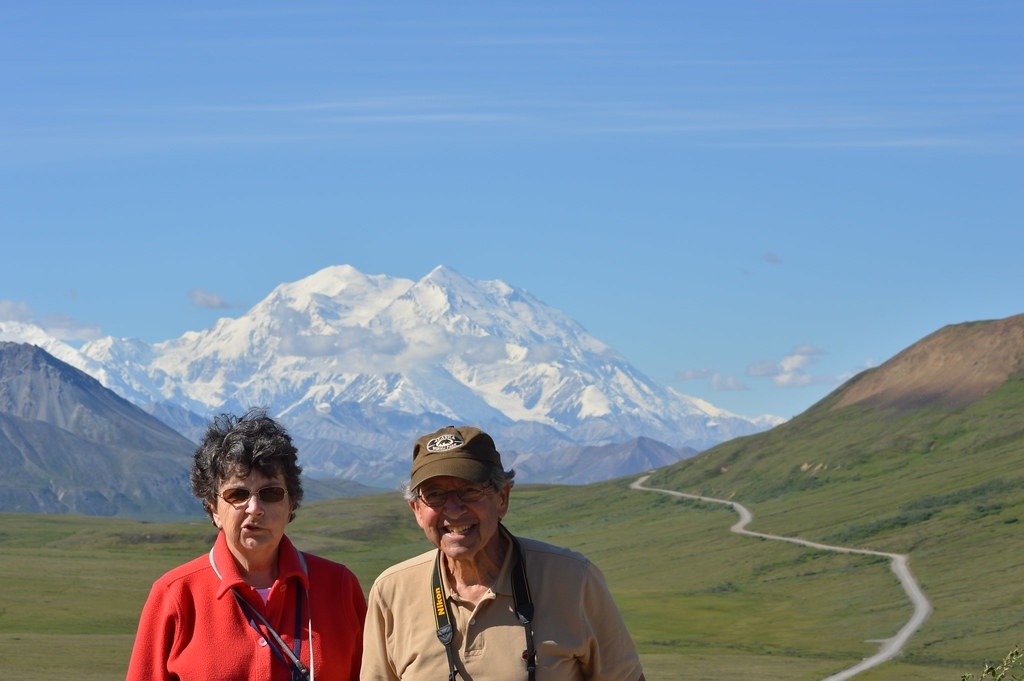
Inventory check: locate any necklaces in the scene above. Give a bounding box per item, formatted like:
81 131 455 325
230 578 314 681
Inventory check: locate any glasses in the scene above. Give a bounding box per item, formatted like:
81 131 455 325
214 485 287 504
416 483 494 507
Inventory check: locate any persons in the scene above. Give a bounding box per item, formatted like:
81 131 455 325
126 405 367 681
360 424 646 681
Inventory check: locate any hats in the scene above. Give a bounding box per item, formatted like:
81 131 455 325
410 426 501 492
